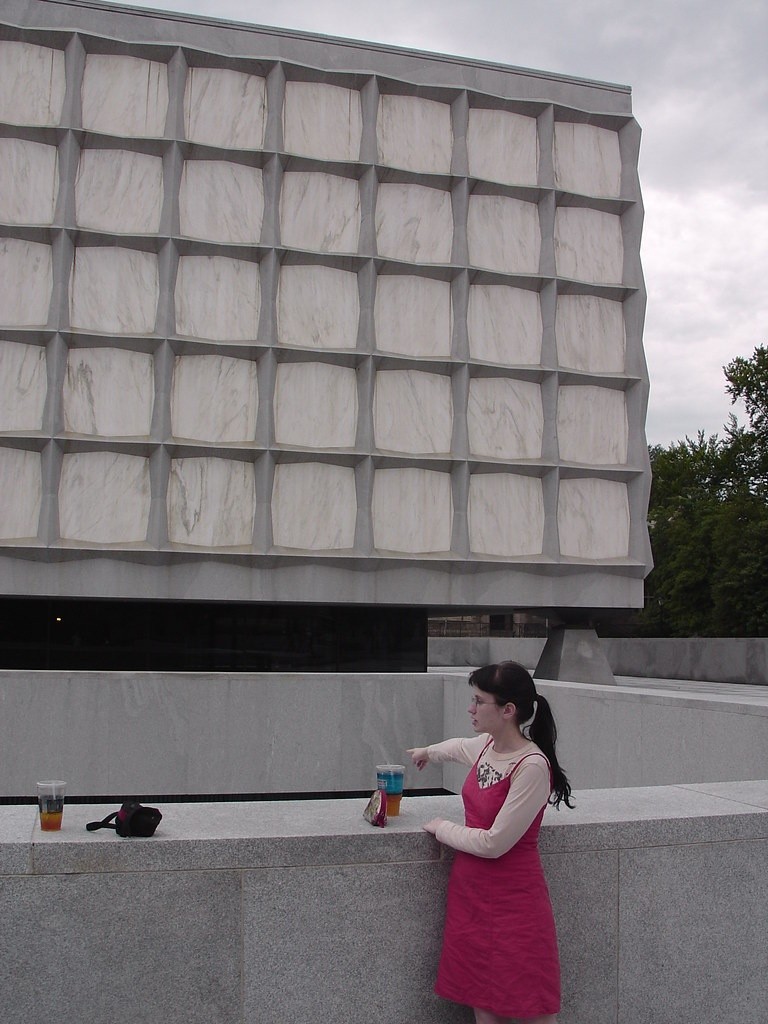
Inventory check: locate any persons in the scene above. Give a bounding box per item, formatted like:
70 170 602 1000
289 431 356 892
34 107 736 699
406 660 576 1024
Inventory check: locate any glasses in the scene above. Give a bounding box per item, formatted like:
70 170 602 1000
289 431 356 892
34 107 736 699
471 695 502 706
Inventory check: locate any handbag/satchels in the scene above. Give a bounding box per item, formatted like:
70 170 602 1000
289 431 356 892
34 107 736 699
115 802 162 837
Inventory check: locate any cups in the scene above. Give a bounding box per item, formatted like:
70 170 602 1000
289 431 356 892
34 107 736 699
36 780 68 832
376 764 405 816
